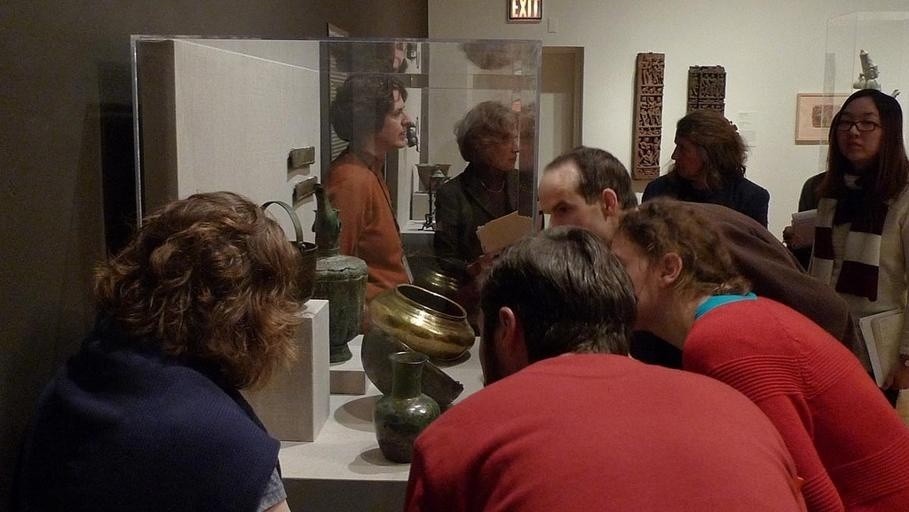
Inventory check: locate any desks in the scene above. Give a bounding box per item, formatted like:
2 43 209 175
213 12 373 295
400 220 436 255
278 327 483 512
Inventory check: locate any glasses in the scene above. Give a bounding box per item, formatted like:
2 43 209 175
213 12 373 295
839 121 882 132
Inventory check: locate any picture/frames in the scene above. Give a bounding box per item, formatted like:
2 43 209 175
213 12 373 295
795 93 850 144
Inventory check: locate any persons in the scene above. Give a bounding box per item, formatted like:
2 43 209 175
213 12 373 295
1 191 294 512
627 105 769 371
538 147 875 387
431 100 536 281
614 196 909 512
789 90 909 409
402 223 808 512
312 72 412 336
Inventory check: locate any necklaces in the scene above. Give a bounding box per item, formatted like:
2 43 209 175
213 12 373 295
477 173 506 195
